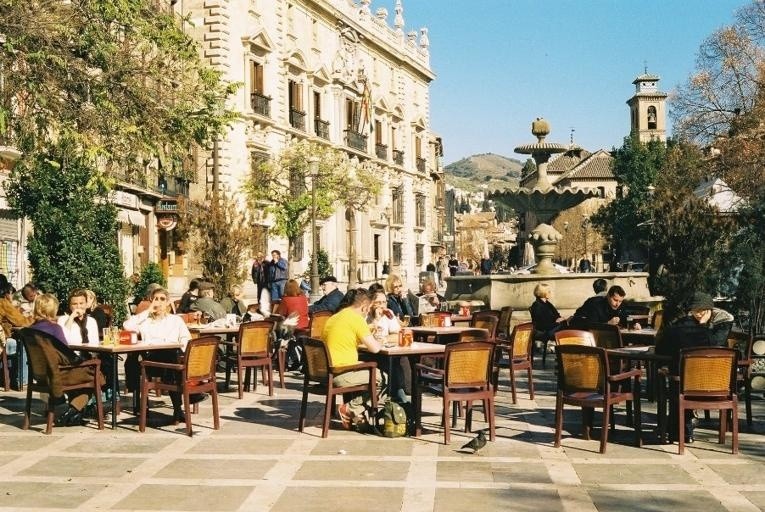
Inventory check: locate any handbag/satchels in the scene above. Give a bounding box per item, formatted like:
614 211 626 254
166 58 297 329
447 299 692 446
373 398 414 437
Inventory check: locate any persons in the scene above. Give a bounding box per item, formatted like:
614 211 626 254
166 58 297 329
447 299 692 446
251 250 459 431
478 254 735 443
0 273 264 427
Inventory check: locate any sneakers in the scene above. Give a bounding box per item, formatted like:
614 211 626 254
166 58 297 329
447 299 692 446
656 430 673 444
685 433 696 443
173 408 187 423
337 403 355 430
188 392 209 404
357 418 370 433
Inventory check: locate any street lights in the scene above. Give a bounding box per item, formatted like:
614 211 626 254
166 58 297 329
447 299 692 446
647 183 655 274
297 155 333 292
580 223 587 257
564 220 571 266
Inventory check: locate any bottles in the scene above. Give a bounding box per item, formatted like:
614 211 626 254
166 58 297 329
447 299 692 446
383 309 394 320
112 326 119 345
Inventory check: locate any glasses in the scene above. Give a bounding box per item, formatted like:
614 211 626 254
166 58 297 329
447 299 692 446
152 296 168 303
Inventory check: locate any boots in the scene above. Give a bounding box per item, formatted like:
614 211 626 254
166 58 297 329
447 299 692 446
53 404 89 426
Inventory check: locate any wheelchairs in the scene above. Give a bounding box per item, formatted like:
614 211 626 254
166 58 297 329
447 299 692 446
267 308 308 370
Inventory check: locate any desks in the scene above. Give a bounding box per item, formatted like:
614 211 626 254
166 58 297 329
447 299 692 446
69 336 183 433
358 336 449 430
183 320 256 393
620 327 663 406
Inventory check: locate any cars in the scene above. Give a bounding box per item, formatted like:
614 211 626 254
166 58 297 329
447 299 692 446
515 262 573 275
456 261 469 272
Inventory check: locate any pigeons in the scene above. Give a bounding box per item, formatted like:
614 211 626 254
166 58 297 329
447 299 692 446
461 429 488 454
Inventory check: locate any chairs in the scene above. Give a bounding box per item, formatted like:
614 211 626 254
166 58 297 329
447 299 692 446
439 328 493 427
138 335 221 437
493 322 536 403
597 324 634 389
557 327 641 392
470 310 501 331
489 305 514 341
310 310 333 340
2 292 311 342
651 310 664 330
726 332 754 423
414 339 497 444
296 333 381 437
269 314 288 390
552 345 645 454
220 320 275 399
0 347 12 392
666 343 744 454
14 326 104 435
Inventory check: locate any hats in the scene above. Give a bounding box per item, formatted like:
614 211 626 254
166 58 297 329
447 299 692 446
319 276 338 286
197 283 216 291
686 292 716 310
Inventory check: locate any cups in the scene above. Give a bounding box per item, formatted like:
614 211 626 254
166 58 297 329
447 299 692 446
444 316 451 327
103 327 111 345
